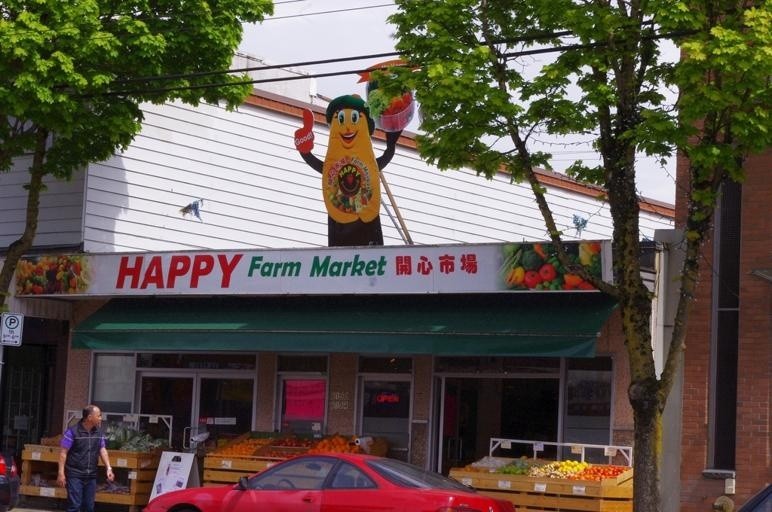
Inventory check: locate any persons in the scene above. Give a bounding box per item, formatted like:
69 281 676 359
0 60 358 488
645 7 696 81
56 405 114 512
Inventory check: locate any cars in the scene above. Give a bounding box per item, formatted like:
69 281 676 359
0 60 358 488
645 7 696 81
0 441 21 512
737 482 772 512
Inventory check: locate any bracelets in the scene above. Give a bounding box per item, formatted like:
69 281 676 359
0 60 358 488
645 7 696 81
106 466 113 470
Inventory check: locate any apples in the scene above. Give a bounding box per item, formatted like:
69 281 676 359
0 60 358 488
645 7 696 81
538 264 558 282
585 465 625 481
524 270 542 288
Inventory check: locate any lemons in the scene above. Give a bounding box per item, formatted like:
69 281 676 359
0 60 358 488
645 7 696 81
553 459 588 473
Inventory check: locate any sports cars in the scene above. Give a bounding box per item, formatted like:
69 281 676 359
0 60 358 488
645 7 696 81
140 450 516 512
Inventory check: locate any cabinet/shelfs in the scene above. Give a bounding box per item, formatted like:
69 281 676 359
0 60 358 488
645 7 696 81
18 443 171 512
450 458 635 512
204 430 362 486
